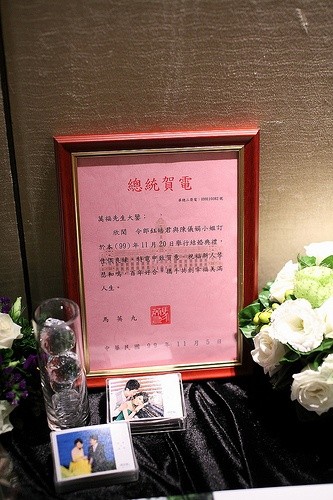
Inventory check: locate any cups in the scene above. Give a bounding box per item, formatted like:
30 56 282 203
33 298 89 432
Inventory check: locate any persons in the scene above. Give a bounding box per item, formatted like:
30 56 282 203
112 391 150 421
87 434 109 474
68 438 92 478
109 380 141 421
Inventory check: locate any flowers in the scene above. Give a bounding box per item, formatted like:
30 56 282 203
0 297 39 435
237 239 333 418
